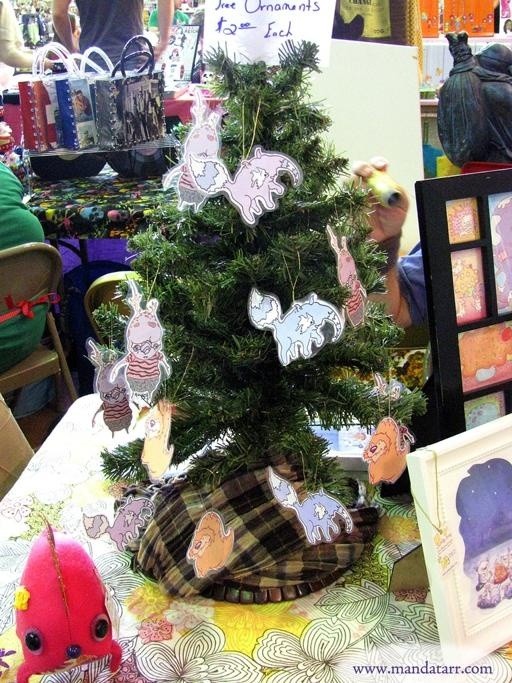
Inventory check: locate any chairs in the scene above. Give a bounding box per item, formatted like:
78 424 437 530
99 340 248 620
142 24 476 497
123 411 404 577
0 241 195 403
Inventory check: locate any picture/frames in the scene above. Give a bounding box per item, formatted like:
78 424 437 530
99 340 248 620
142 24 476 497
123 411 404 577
405 411 512 673
415 168 512 437
168 25 201 82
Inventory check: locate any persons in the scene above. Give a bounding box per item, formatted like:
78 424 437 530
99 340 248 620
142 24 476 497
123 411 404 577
12 514 123 683
1 161 55 421
331 153 431 331
50 0 176 72
503 19 512 34
1 0 53 94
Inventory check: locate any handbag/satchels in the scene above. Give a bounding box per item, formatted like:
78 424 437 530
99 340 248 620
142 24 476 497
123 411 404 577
17 42 79 154
55 46 115 148
96 35 167 148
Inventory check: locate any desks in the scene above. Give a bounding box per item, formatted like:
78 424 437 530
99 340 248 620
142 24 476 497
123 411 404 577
162 82 231 124
0 391 512 683
421 33 512 144
19 135 194 347
